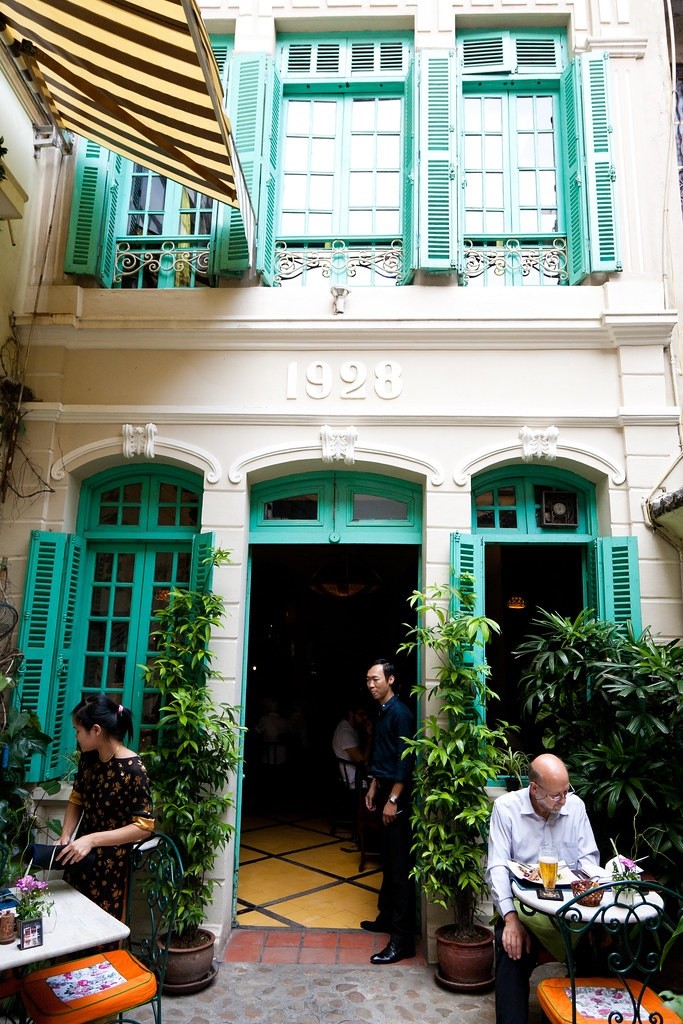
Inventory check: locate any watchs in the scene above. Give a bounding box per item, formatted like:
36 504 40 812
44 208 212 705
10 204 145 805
388 795 398 803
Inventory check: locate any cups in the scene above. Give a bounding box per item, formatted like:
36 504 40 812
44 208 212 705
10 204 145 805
538 846 559 897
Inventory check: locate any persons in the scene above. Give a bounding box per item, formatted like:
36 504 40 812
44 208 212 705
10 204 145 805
360 658 416 965
254 698 374 790
484 753 600 1024
52 694 155 966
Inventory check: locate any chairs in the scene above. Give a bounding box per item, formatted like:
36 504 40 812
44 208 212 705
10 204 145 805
257 733 381 872
536 880 683 1024
19 833 185 1024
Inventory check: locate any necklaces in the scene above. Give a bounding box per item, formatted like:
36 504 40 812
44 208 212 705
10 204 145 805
99 741 122 763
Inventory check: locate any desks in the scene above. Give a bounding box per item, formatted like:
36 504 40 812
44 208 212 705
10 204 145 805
0 879 131 1024
514 869 666 978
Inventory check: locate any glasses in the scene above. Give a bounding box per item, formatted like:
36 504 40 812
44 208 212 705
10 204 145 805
355 711 369 717
532 780 576 801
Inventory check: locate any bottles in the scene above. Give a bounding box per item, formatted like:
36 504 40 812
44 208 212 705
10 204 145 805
1 910 14 939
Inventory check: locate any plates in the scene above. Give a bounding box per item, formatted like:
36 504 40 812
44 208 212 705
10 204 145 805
505 858 581 885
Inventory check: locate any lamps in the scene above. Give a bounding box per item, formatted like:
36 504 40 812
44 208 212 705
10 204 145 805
507 595 526 609
307 549 378 598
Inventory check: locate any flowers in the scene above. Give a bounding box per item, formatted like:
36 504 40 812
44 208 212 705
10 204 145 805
15 874 54 921
612 858 641 905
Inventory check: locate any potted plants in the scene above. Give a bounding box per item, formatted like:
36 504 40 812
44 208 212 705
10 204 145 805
394 566 522 984
494 746 537 791
136 544 249 984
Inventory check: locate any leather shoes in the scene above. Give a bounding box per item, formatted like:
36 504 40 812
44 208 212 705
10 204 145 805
370 941 417 964
360 917 392 933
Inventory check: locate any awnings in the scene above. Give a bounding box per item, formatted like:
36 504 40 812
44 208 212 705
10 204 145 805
0 0 257 279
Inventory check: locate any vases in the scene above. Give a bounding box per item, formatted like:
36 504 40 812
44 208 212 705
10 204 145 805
611 887 640 905
16 917 42 949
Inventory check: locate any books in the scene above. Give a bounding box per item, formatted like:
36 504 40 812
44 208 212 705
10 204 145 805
505 853 644 886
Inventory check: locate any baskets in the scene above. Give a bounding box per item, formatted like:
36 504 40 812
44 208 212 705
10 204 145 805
570 876 604 907
